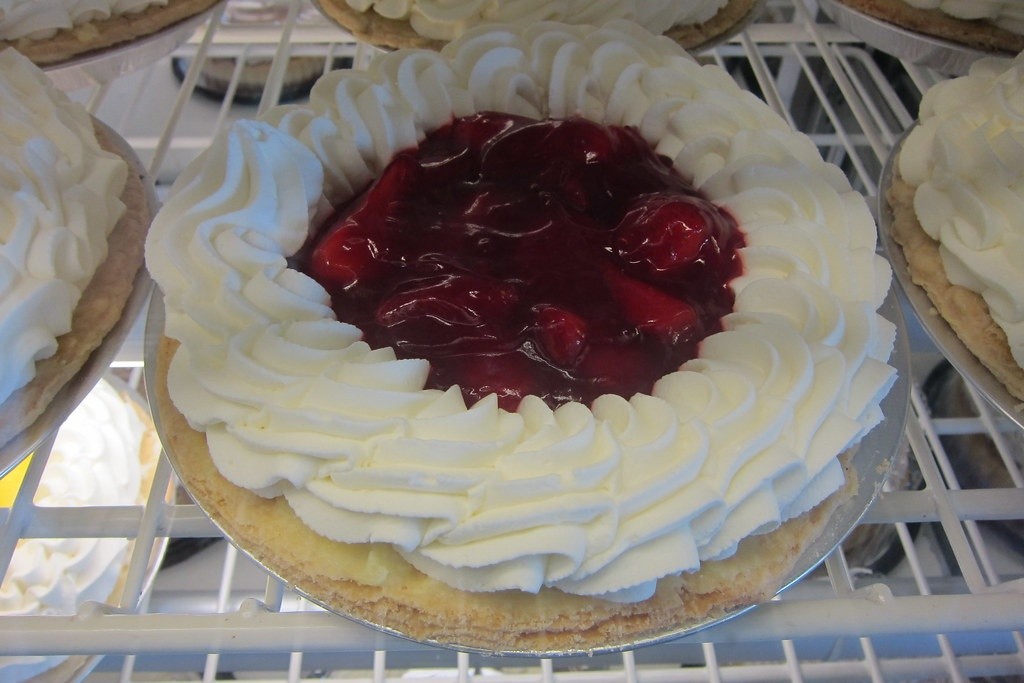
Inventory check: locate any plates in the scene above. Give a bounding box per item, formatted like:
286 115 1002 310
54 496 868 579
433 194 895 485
920 356 1024 559
68 369 179 683
39 0 225 94
310 0 767 57
852 474 929 579
142 284 913 658
0 113 162 482
877 118 1024 430
819 0 1016 77
170 56 354 106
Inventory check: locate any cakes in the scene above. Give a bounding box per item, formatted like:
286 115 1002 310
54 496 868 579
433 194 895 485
0 0 1024 652
